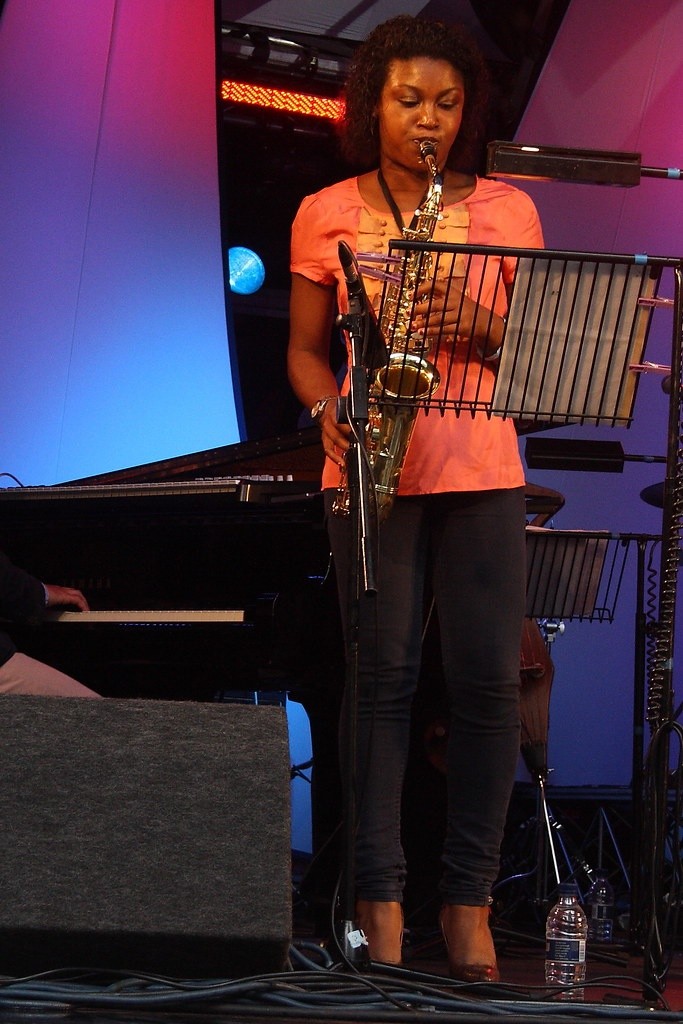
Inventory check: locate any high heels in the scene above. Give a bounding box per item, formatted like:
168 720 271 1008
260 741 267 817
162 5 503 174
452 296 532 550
437 902 501 984
354 899 405 970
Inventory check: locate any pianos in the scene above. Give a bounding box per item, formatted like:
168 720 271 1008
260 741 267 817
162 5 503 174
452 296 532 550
0 471 324 703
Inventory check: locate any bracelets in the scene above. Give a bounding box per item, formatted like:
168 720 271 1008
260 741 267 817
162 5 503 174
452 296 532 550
475 317 507 362
41 583 49 604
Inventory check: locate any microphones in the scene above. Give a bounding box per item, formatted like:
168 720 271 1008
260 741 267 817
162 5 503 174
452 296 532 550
337 243 389 371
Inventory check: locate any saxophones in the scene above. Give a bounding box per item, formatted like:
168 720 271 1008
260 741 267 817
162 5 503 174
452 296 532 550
372 140 444 531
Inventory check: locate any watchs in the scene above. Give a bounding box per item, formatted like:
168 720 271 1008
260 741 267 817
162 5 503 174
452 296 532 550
309 393 338 426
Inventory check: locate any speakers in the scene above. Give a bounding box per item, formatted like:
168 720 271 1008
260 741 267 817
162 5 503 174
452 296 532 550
1 692 293 978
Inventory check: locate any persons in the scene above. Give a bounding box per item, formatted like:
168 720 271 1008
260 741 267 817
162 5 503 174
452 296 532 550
0 546 106 698
284 12 546 994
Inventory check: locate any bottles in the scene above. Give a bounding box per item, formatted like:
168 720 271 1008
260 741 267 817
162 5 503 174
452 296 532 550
587 868 613 944
545 881 588 1003
